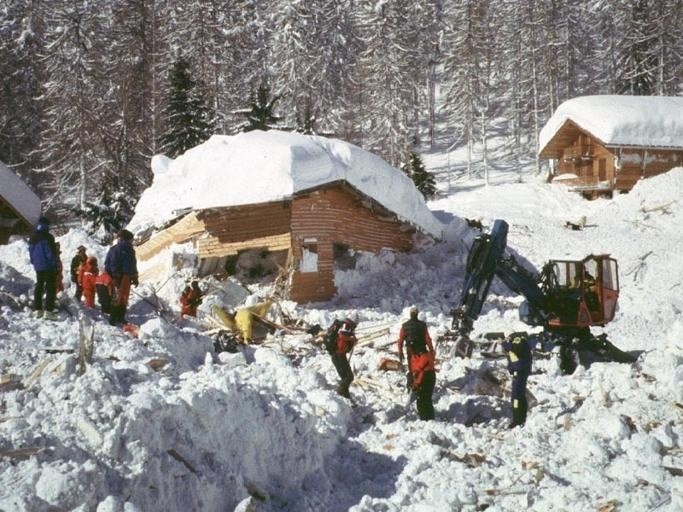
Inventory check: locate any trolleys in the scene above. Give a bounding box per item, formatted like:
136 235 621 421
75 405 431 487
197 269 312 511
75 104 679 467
568 172 613 201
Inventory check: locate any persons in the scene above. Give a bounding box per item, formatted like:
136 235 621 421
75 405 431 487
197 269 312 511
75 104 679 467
501 333 533 429
404 335 435 420
108 230 140 326
52 241 64 295
566 264 595 309
180 282 202 321
29 217 65 321
330 312 360 398
96 272 112 314
398 308 437 395
70 245 87 302
78 257 98 309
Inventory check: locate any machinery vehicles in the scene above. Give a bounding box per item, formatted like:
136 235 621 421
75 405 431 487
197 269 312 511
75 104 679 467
434 218 640 372
